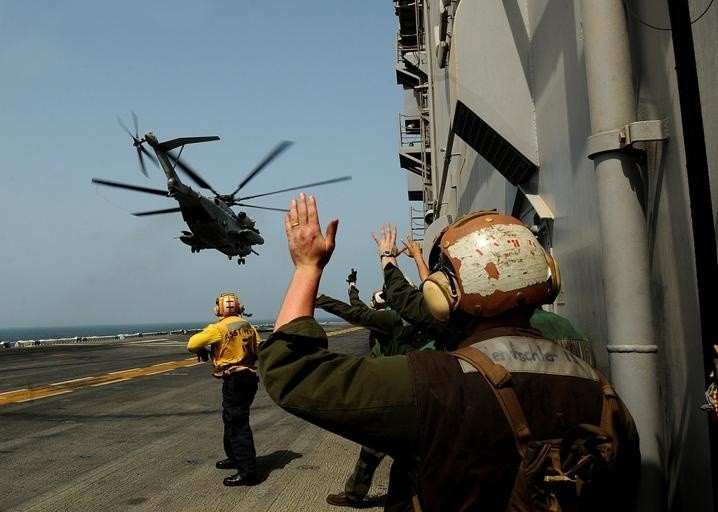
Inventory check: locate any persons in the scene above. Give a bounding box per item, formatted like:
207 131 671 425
256 191 645 512
186 292 264 487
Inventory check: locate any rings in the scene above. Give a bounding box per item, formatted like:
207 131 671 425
290 222 300 228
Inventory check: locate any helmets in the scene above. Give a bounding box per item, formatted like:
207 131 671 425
370 290 387 309
423 210 561 322
213 293 245 316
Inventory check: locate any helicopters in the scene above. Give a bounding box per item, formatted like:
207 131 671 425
90 114 351 264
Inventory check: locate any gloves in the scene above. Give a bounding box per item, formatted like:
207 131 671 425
346 268 357 283
195 347 211 362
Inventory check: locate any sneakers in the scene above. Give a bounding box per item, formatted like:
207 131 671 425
326 492 366 509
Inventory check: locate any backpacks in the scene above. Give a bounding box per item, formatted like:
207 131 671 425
452 345 618 512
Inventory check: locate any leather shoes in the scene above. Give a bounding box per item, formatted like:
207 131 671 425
223 473 246 486
216 458 238 469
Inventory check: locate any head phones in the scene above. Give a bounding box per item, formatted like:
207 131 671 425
214 303 245 316
421 252 561 323
370 299 376 308
